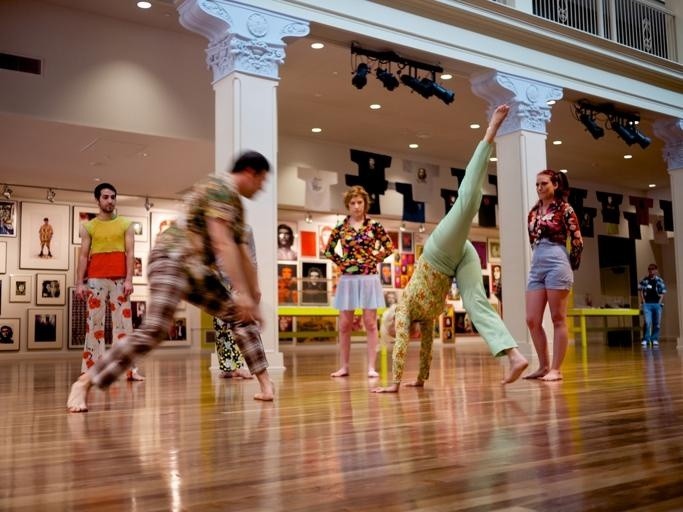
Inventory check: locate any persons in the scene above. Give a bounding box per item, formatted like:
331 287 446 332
211 222 257 381
637 263 669 348
381 264 392 284
368 103 530 394
415 167 428 183
65 146 276 417
368 158 376 170
176 317 187 338
277 224 335 337
36 216 56 262
16 282 25 295
385 291 396 306
491 264 501 299
137 302 146 318
0 326 14 344
43 282 51 297
73 181 148 385
50 281 60 297
322 183 396 378
520 167 586 384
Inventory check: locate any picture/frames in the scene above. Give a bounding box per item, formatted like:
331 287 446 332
0 195 191 352
276 217 504 300
453 310 480 336
442 304 457 344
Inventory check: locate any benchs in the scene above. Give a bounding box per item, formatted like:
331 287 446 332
277 302 388 348
566 305 654 350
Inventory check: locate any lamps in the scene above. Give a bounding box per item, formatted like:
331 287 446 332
333 42 464 113
569 90 660 154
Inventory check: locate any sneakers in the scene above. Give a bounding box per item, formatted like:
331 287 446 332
641 340 647 345
653 340 659 345
